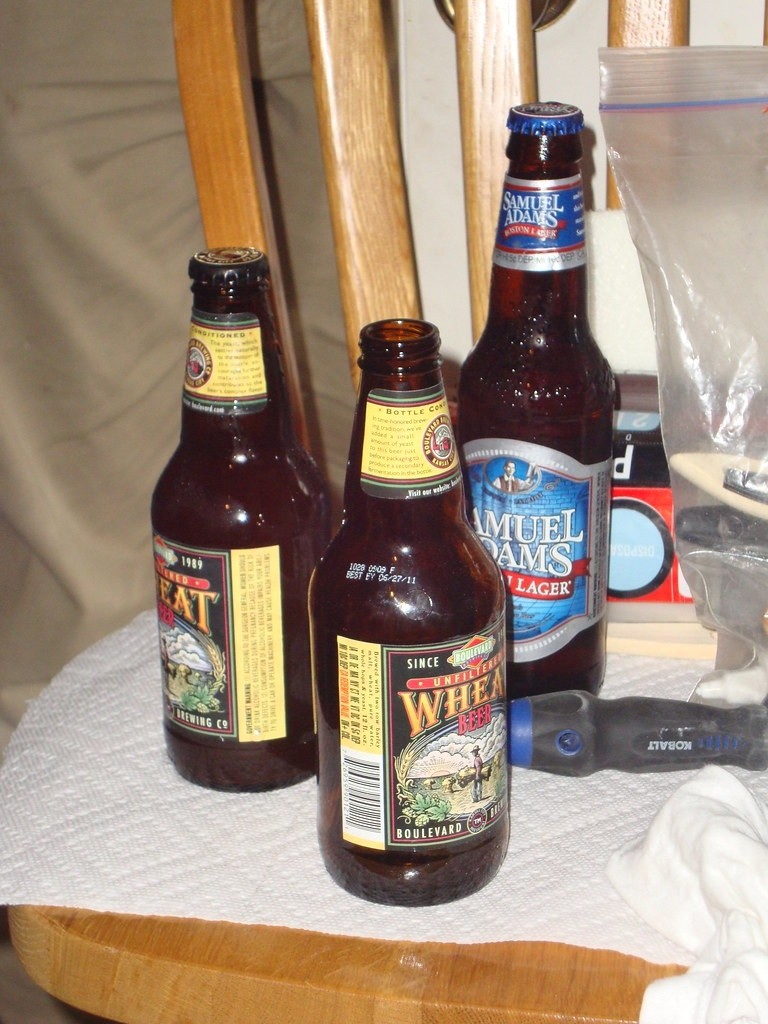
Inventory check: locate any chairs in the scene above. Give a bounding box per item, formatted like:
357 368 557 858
0 0 767 1024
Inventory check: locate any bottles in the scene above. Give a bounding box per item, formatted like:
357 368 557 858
454 102 615 700
307 319 508 906
149 247 327 794
